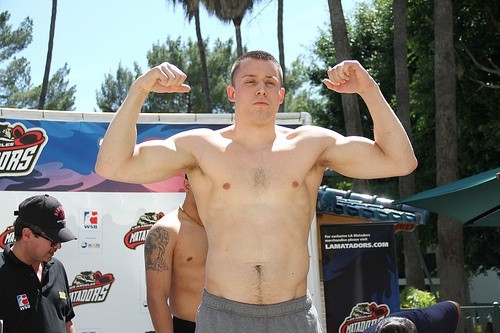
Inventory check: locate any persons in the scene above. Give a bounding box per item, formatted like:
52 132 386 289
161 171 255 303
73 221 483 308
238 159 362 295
94 49 417 333
361 300 460 333
1 195 77 332
144 173 209 332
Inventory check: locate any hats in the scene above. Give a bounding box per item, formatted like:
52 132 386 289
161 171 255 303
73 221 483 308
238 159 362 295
14 194 77 242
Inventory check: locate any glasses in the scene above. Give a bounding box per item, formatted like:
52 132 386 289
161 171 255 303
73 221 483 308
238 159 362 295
31 229 58 247
